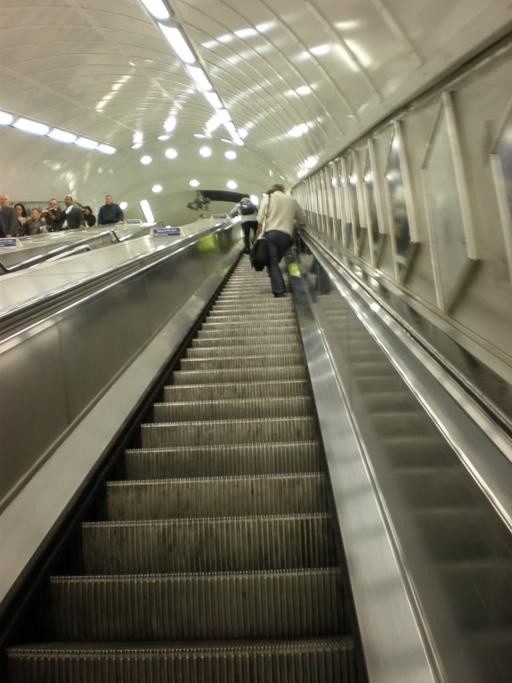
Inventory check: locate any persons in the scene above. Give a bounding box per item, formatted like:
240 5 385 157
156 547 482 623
228 195 259 255
97 194 123 226
256 184 306 298
0 193 96 239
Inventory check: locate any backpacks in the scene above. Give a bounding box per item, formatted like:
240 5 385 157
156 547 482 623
240 197 255 215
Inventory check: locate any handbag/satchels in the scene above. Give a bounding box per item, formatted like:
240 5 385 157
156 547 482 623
249 239 270 271
